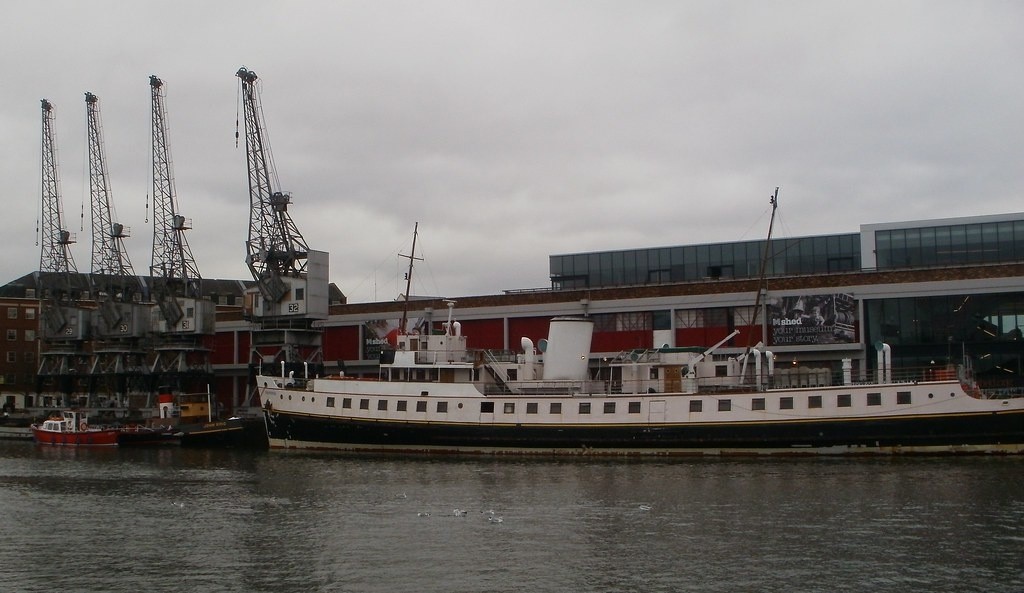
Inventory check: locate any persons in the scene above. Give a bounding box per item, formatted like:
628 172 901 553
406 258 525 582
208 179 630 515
383 318 421 347
794 306 825 325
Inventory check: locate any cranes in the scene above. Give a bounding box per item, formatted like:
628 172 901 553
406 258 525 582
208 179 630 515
235 68 329 419
36 98 100 381
81 92 155 392
146 75 223 410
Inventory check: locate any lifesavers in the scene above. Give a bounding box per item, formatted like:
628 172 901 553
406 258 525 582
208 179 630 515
81 423 87 431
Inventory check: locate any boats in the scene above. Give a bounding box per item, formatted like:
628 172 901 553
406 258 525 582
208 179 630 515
173 392 245 442
30 411 122 447
121 425 184 444
256 302 1024 461
